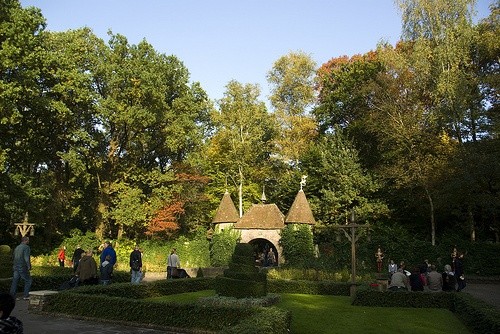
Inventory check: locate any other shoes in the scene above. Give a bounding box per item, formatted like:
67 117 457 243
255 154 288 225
24 297 30 300
15 297 20 301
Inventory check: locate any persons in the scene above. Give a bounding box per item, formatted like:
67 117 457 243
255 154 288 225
10 236 33 300
267 248 275 266
71 244 84 273
167 248 180 280
0 292 16 319
100 241 116 272
388 248 465 293
375 247 385 273
101 255 113 285
75 250 97 285
130 245 142 283
258 249 265 268
58 246 66 267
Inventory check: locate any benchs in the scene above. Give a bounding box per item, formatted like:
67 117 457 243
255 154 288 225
29 290 60 312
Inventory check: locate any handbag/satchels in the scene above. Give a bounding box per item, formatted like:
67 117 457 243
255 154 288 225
171 268 178 275
69 276 78 287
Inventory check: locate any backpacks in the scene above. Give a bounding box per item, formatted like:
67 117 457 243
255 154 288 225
132 252 140 271
